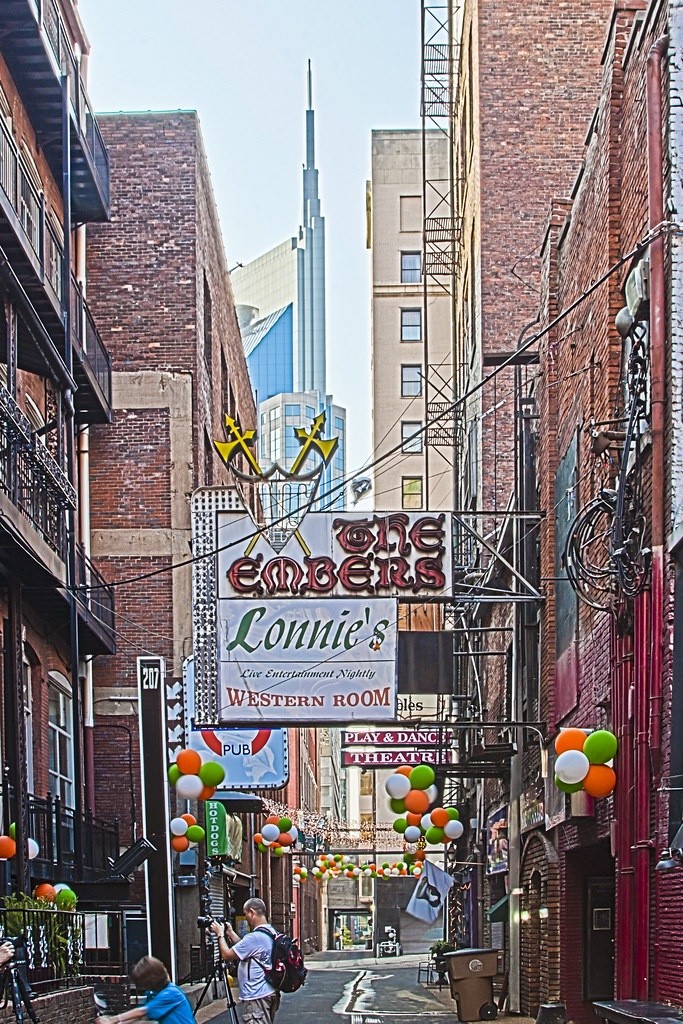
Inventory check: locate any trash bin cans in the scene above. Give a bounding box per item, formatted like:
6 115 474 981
443 948 500 1022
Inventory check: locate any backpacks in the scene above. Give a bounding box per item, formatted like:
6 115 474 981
248 927 308 993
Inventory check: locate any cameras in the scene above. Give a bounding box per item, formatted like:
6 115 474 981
0 935 29 947
197 916 227 933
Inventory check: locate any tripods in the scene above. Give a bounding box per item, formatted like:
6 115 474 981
0 957 37 1024
193 953 240 1024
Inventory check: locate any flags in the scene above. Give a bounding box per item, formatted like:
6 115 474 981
405 861 455 925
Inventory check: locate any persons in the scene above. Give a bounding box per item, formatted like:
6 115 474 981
210 898 280 1024
0 941 15 1002
96 954 197 1024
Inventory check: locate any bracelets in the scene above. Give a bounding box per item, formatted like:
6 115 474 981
218 935 224 939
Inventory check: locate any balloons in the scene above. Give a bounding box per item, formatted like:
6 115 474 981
36 883 76 908
170 814 205 852
555 727 617 796
312 854 377 881
169 749 224 800
379 861 423 880
254 816 297 856
385 763 463 843
0 822 39 859
293 867 307 883
